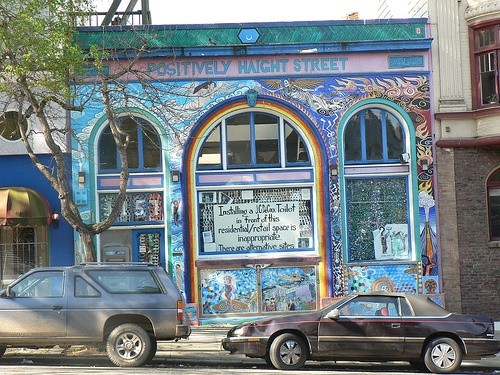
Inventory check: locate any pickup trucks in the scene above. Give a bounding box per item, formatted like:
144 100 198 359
0 259 193 367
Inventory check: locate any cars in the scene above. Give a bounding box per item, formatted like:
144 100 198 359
220 292 500 375
